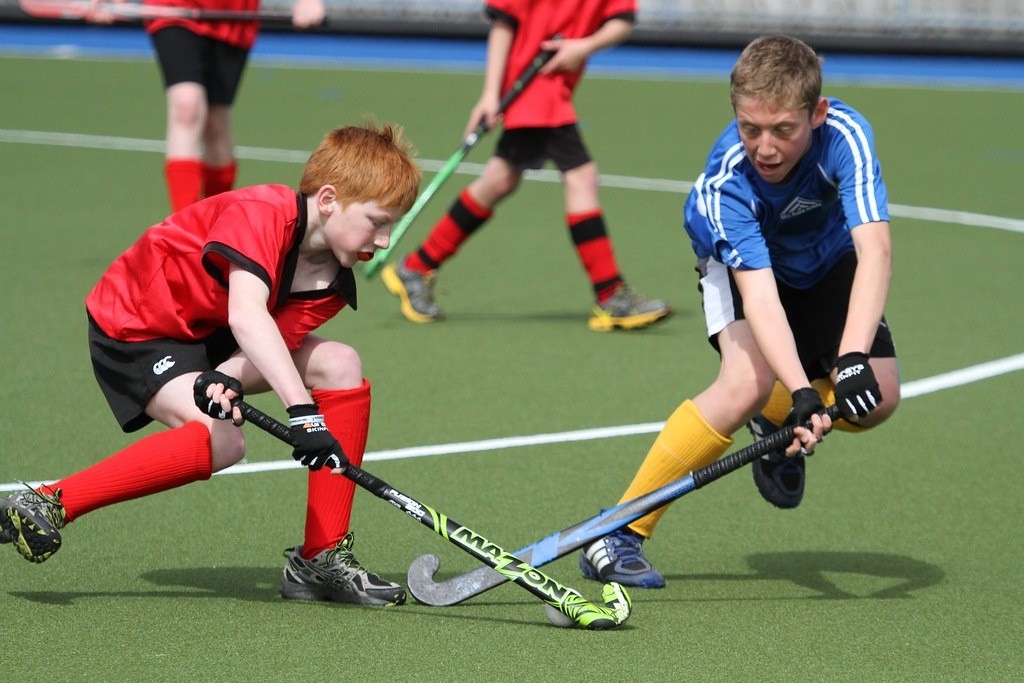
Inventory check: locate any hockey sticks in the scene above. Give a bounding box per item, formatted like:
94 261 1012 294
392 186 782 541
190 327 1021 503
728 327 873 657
405 402 843 607
20 0 331 27
222 392 634 628
353 33 566 282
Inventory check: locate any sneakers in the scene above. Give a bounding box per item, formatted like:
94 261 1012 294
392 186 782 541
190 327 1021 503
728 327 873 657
1 478 66 564
579 525 666 588
746 411 805 509
381 251 441 322
280 531 406 607
588 284 671 331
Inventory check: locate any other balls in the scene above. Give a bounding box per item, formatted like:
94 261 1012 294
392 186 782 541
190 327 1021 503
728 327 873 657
544 588 583 628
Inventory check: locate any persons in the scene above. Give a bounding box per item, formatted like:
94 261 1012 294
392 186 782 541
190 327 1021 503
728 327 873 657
1 119 420 608
578 33 901 589
147 0 261 216
379 1 670 332
